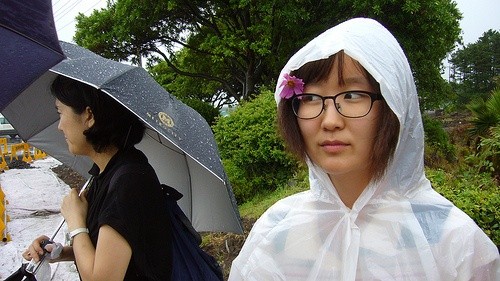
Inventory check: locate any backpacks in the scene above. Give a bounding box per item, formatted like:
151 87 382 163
161 180 224 281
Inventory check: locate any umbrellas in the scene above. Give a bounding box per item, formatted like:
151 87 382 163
0 40 245 281
0 0 68 112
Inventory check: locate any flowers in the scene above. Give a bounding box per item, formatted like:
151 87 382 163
278 73 305 100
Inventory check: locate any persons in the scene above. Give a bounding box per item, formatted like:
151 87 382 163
22 74 177 281
227 17 500 281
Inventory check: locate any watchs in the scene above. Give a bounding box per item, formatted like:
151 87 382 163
65 228 89 247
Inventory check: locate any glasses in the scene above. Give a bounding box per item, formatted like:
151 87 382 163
291 89 382 119
